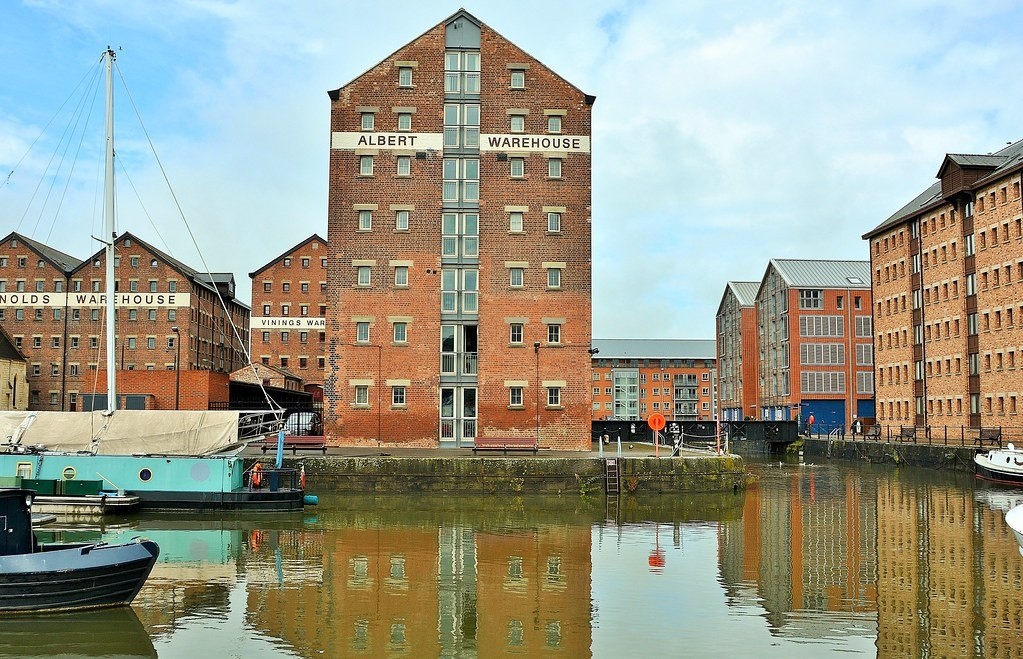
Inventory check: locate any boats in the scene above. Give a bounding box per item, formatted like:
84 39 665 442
1004 503 1023 558
0 488 160 615
972 442 1023 489
972 490 1023 512
0 475 141 507
32 530 324 592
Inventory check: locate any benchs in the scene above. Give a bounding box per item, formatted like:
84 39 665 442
974 429 1000 446
893 428 915 442
261 436 326 454
864 427 882 440
472 437 538 455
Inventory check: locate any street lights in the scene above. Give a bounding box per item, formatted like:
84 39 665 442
172 325 179 410
534 342 540 445
63 269 73 410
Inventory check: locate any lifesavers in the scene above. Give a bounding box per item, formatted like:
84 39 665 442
251 528 261 553
301 473 306 490
252 463 263 486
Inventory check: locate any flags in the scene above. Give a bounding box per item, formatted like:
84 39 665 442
301 466 306 488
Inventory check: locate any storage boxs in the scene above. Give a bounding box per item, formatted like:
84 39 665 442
20 480 103 495
34 531 102 543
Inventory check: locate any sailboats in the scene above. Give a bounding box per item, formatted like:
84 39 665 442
0 44 319 512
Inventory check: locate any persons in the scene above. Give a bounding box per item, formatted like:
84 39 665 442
310 414 320 435
